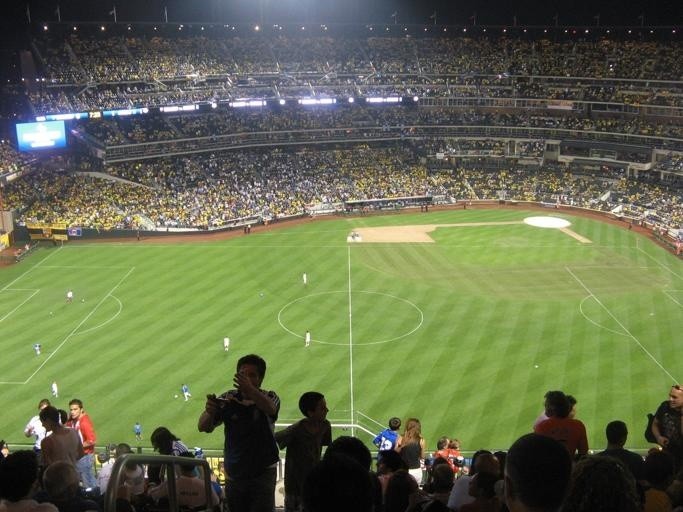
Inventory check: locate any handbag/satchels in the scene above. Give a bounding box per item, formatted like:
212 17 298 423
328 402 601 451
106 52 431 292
644 413 664 446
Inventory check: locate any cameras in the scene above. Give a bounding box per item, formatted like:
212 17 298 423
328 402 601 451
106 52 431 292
665 441 672 448
214 398 230 412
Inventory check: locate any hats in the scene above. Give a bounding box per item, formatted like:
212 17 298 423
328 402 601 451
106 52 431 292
177 451 197 478
